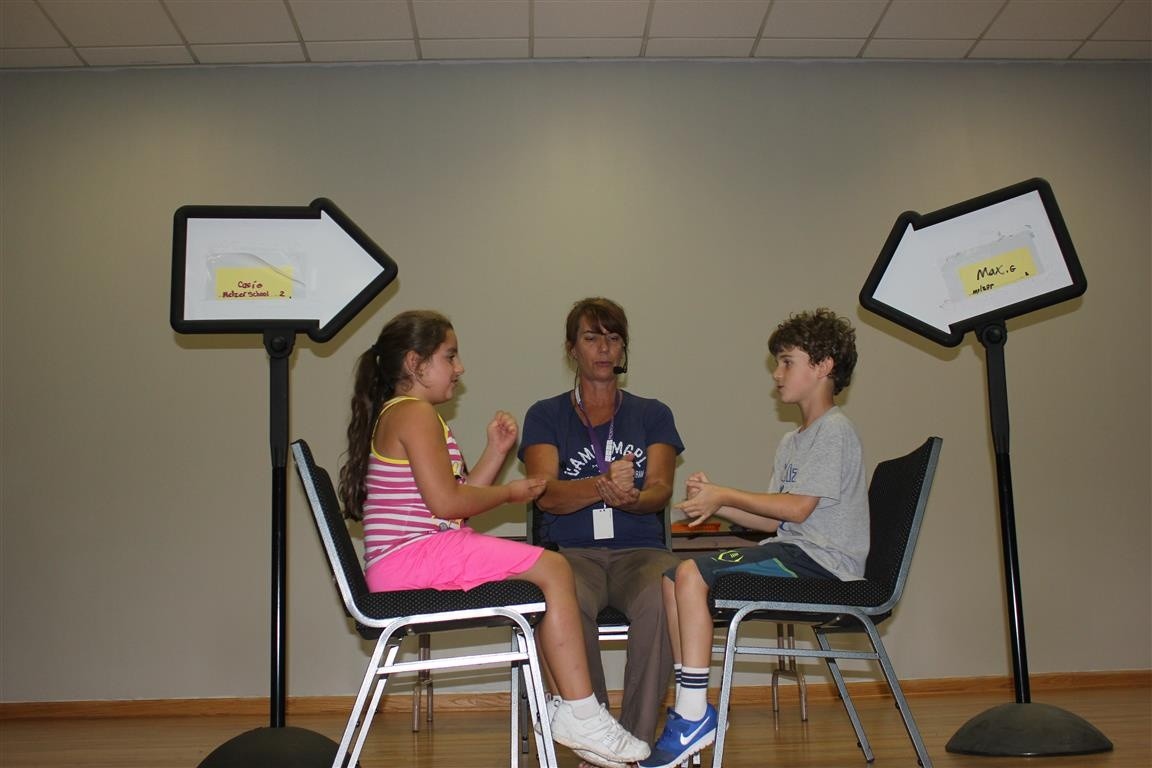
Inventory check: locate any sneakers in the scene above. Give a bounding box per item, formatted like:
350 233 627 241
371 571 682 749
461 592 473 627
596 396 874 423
534 693 632 768
550 700 652 763
635 703 730 768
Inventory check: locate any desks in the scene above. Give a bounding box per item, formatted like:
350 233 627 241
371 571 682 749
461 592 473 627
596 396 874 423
406 524 808 732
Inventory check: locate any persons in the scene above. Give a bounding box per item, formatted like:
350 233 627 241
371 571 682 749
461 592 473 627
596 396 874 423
634 306 873 768
517 297 684 768
338 311 651 768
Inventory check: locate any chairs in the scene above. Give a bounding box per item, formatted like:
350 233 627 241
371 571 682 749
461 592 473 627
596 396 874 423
518 453 678 752
288 438 561 768
705 437 946 768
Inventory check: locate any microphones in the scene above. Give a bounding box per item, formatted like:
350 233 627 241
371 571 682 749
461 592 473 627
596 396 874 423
612 344 630 374
